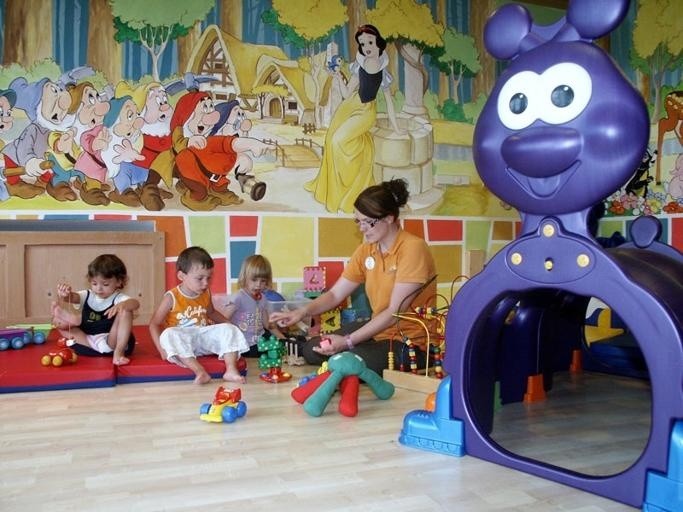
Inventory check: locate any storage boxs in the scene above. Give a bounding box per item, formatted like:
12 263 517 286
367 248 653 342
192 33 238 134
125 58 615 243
267 294 313 328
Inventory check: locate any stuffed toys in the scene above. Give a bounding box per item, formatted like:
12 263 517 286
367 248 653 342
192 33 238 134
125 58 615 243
288 350 396 418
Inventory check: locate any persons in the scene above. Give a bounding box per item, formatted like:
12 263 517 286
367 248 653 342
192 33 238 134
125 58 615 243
266 177 439 394
147 246 249 387
303 22 408 213
221 253 308 358
46 252 141 367
0 73 280 211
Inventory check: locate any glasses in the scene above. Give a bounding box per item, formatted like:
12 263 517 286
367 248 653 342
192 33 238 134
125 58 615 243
354 214 380 229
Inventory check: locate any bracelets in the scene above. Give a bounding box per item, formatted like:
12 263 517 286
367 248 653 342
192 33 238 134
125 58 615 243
343 333 355 351
